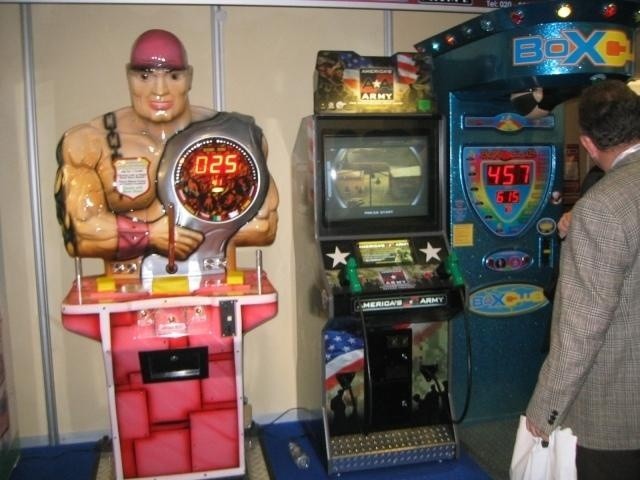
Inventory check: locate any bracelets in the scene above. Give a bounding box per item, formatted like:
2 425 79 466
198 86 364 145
117 214 149 256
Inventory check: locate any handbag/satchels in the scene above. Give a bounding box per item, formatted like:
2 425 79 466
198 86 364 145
508 412 581 480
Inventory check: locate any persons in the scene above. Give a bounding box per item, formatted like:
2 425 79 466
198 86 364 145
54 28 280 270
331 389 347 418
412 382 448 412
524 79 640 480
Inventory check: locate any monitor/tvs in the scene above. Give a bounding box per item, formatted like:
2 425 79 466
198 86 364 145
313 111 447 243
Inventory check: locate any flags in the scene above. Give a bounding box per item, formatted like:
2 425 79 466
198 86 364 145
322 323 443 393
